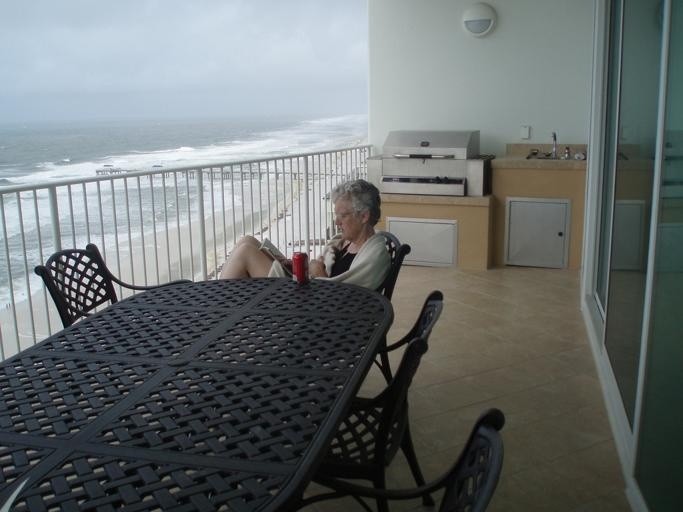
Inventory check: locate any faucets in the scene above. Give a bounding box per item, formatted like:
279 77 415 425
551 132 556 158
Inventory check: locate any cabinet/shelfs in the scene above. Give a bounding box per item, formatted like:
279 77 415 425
374 193 494 270
491 159 587 271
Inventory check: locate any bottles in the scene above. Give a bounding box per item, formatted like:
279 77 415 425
292 252 309 283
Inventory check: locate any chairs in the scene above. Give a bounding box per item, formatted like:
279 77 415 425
33 243 191 330
376 231 411 385
311 289 443 512
293 408 506 512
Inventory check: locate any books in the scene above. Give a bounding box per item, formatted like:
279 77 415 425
258 237 287 265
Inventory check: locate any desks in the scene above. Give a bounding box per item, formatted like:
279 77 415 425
0 279 393 512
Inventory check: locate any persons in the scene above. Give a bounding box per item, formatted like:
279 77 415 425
220 179 392 292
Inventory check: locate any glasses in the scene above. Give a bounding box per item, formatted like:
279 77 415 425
328 212 353 220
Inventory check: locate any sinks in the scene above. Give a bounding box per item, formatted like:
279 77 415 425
525 154 575 161
